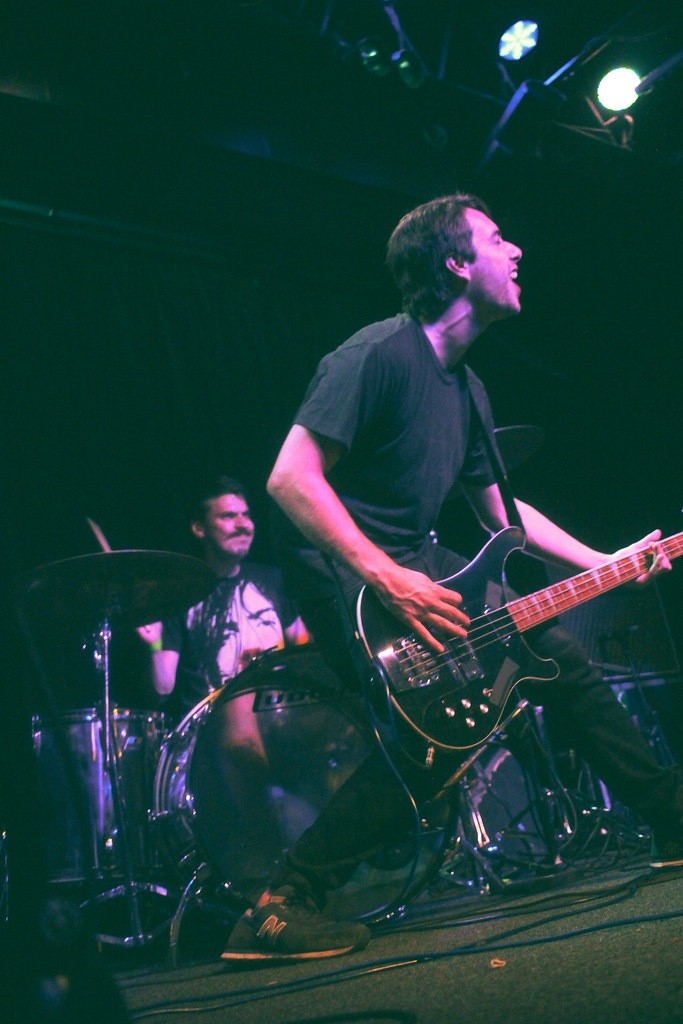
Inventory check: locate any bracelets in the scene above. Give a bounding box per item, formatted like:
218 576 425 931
144 640 162 653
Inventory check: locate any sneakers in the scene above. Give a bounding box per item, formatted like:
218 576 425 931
220 901 369 963
648 829 683 868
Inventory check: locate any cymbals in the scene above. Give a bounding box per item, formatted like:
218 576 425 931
443 424 547 505
6 544 220 638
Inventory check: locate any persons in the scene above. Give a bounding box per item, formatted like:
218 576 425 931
219 193 683 959
133 475 310 751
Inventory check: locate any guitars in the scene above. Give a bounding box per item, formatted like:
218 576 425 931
347 523 683 777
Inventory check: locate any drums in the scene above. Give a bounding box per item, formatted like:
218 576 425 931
154 641 467 932
595 669 683 771
29 704 172 896
